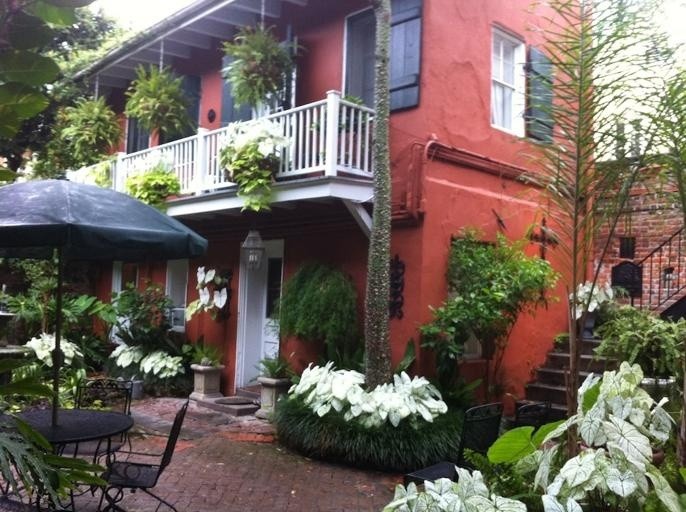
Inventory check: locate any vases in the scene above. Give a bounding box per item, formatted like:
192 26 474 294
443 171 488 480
577 312 596 340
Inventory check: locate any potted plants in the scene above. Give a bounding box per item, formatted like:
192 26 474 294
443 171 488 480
61 22 305 165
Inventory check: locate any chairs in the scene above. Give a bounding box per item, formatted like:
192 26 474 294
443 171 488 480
54 377 192 511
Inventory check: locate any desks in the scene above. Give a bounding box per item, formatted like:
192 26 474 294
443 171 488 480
13 408 134 511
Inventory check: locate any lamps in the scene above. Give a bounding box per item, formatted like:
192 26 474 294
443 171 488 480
241 206 265 271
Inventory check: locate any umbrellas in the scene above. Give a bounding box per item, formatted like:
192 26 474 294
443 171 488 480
0 176 209 422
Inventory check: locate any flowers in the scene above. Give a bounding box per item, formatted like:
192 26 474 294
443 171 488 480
270 362 686 512
565 280 612 320
219 116 292 211
31 263 231 377
